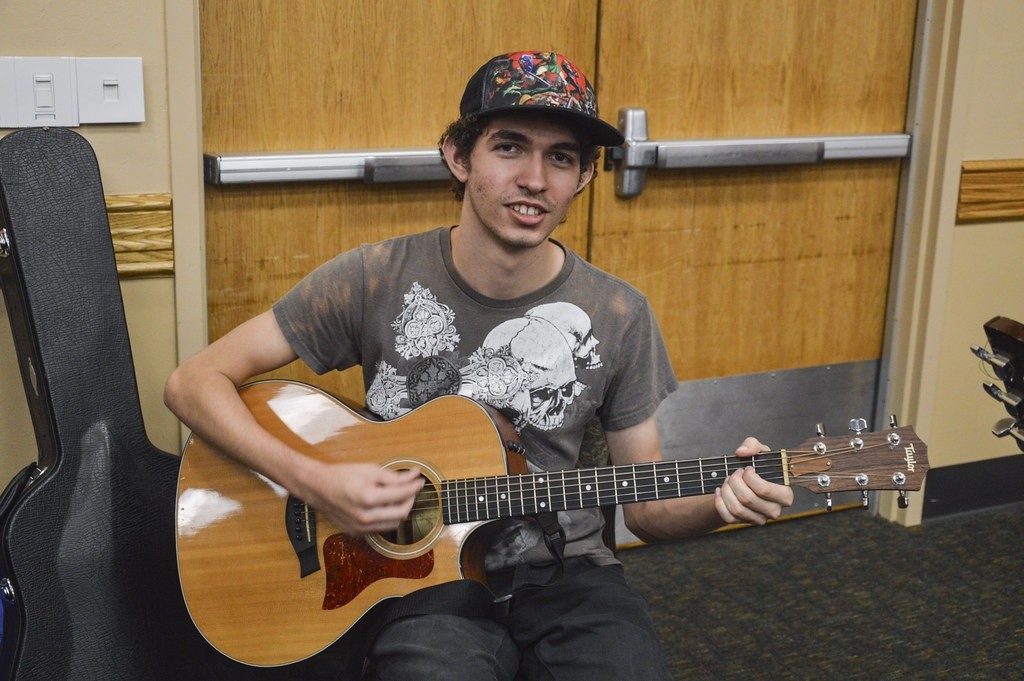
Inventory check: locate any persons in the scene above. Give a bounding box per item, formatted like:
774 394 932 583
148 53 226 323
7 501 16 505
165 52 795 680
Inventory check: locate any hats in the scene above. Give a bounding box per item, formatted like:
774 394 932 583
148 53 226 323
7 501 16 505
454 51 626 148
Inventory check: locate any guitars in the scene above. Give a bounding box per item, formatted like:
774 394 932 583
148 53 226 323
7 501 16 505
170 378 932 670
